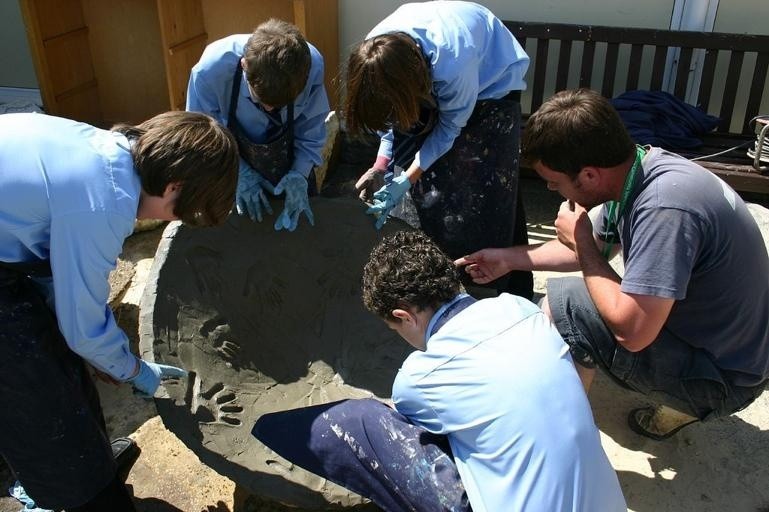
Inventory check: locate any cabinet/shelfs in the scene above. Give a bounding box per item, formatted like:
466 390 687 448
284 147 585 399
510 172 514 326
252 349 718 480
19 1 340 122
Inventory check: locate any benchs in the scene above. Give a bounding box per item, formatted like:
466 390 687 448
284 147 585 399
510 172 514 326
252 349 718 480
498 19 768 198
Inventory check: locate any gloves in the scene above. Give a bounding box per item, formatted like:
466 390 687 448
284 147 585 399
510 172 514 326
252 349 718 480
7 479 39 510
354 169 413 231
127 358 188 402
235 153 274 223
272 170 316 233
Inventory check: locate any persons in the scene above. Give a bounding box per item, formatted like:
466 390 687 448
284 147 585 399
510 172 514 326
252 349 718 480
1 111 237 512
336 0 533 300
186 18 331 234
309 227 630 512
453 86 769 439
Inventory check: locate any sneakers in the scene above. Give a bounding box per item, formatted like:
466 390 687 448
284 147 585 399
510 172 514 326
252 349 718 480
110 434 138 468
627 401 704 442
